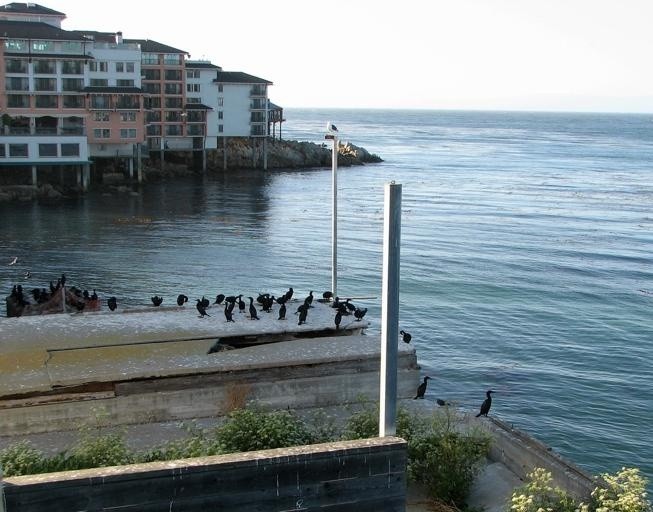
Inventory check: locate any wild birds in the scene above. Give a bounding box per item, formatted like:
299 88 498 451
194 296 211 318
214 287 296 322
107 296 118 312
399 329 412 345
475 390 496 418
150 295 163 307
322 291 333 299
295 290 313 326
332 296 368 328
176 294 189 306
11 273 98 313
413 375 434 402
